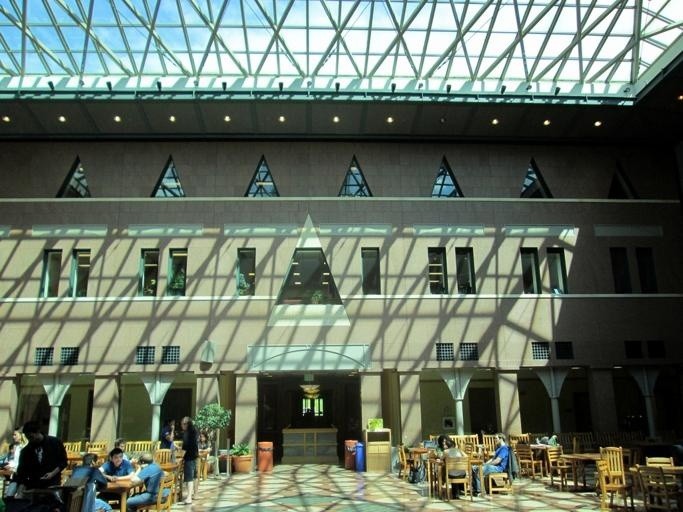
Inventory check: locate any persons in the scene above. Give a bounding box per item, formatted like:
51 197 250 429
175 416 199 505
472 433 509 497
304 409 310 416
0 418 179 512
436 435 448 452
440 440 468 499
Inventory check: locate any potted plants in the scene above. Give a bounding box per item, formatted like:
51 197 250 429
229 440 253 474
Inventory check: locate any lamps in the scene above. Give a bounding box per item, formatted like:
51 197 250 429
298 373 321 400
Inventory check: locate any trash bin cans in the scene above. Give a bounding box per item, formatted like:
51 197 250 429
344 440 357 469
257 441 274 471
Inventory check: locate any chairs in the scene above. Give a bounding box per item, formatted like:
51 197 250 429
0 439 209 512
397 433 683 512
